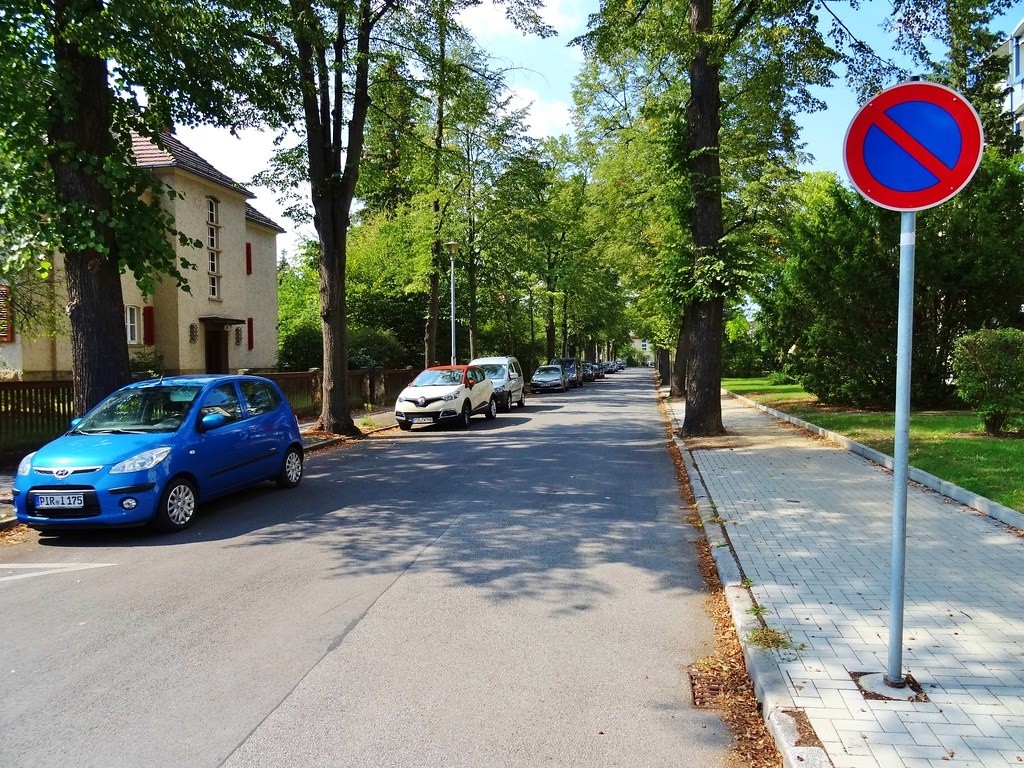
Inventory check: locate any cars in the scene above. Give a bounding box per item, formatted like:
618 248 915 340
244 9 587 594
592 363 605 380
600 360 625 374
582 362 595 383
10 373 302 533
395 364 497 431
530 366 571 394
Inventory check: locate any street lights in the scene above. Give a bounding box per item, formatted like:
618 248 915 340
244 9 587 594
443 239 461 366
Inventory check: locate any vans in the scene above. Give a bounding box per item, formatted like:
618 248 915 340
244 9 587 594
467 355 525 412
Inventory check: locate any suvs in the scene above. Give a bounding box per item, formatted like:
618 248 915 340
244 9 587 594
551 358 583 388
